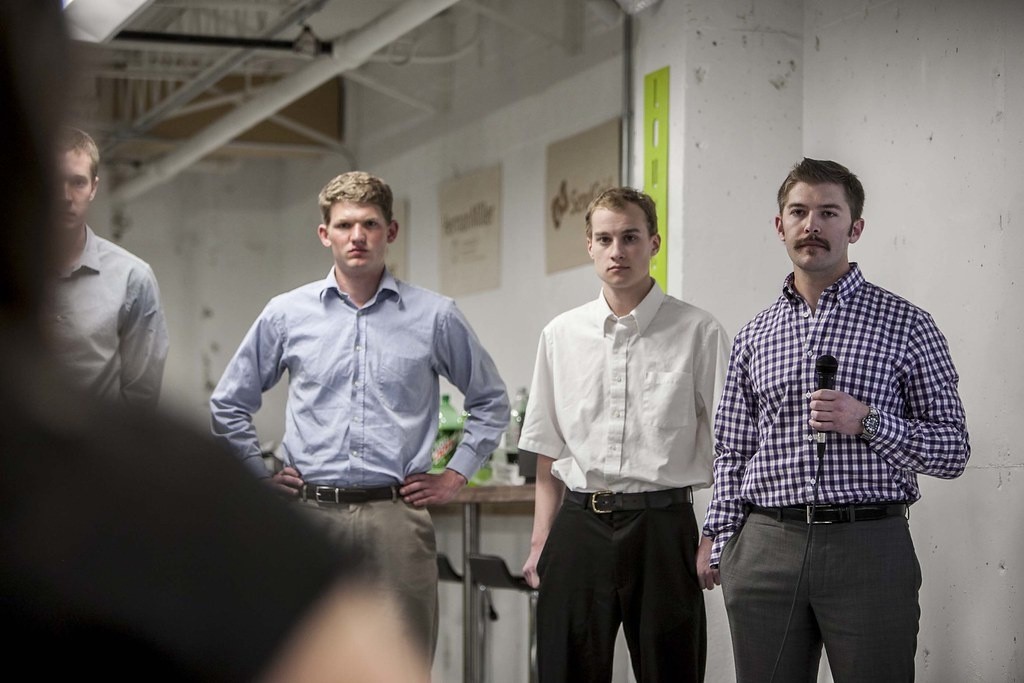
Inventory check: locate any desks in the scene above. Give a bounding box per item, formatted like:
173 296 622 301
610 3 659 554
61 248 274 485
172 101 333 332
428 479 571 683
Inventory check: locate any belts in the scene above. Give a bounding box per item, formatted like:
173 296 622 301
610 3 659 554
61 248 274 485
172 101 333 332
566 488 692 514
748 503 907 525
297 485 405 505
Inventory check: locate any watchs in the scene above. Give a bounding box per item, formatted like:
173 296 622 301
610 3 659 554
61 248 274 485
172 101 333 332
859 405 880 442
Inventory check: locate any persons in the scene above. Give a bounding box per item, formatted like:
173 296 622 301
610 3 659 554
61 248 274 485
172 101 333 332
44 127 167 415
209 172 512 672
0 0 423 683
694 156 970 683
516 188 732 683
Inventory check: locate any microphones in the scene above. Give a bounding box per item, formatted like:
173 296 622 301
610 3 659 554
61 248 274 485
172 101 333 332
817 355 839 457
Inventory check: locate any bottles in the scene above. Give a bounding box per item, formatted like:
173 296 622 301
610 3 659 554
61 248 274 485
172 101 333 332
426 395 463 473
461 387 531 484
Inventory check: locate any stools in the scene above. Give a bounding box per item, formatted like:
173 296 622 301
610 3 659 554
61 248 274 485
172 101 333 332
468 544 540 683
437 551 464 683
